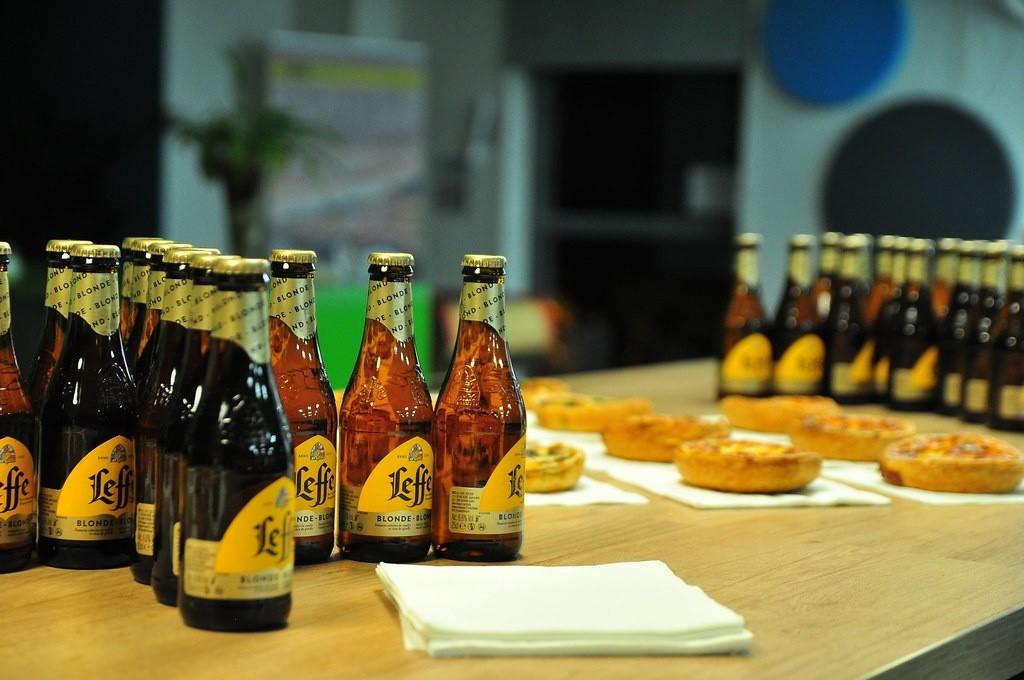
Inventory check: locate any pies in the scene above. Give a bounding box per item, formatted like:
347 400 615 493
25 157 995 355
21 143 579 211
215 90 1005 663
520 377 1023 494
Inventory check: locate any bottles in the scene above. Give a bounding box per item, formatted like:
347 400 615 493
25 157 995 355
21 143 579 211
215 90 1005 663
432 255 528 563
340 252 434 564
716 232 1023 434
0 237 339 632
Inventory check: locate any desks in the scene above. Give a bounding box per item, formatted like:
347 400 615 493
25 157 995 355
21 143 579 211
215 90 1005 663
0 359 1024 680
